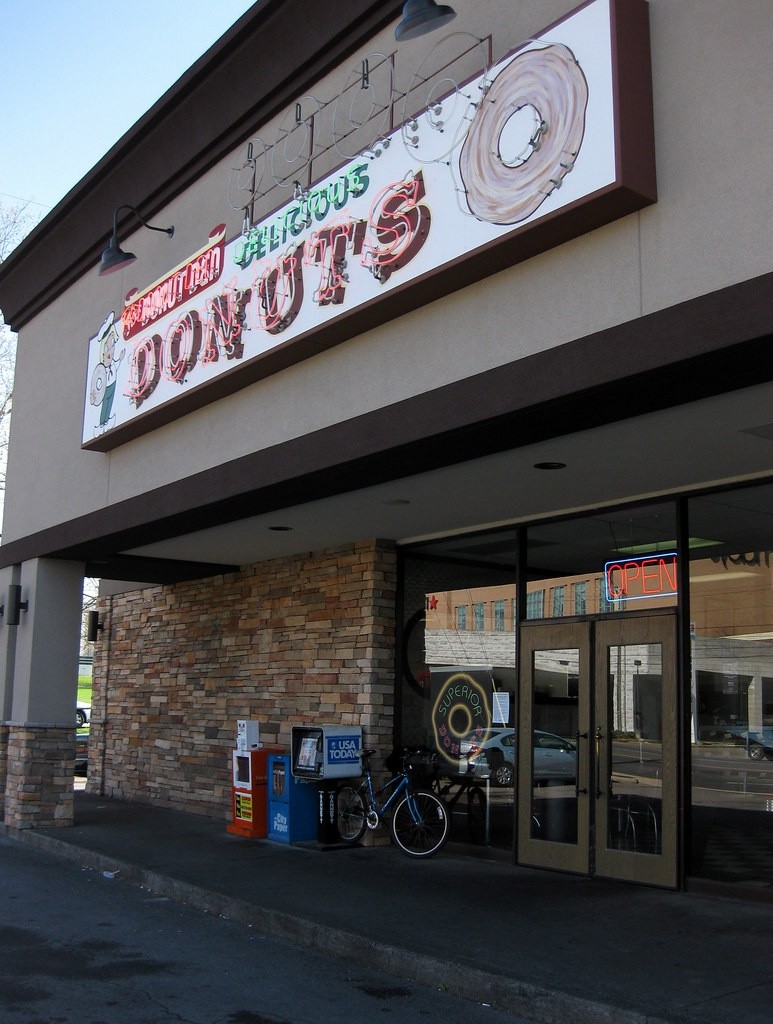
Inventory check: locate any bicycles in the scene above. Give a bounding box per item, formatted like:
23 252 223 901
336 748 452 858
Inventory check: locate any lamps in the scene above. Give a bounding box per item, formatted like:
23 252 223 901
5 585 29 626
98 204 175 277
395 0 457 42
88 611 104 642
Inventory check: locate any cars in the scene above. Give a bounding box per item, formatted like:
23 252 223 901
459 729 579 785
75 734 89 772
730 724 773 762
76 700 91 728
